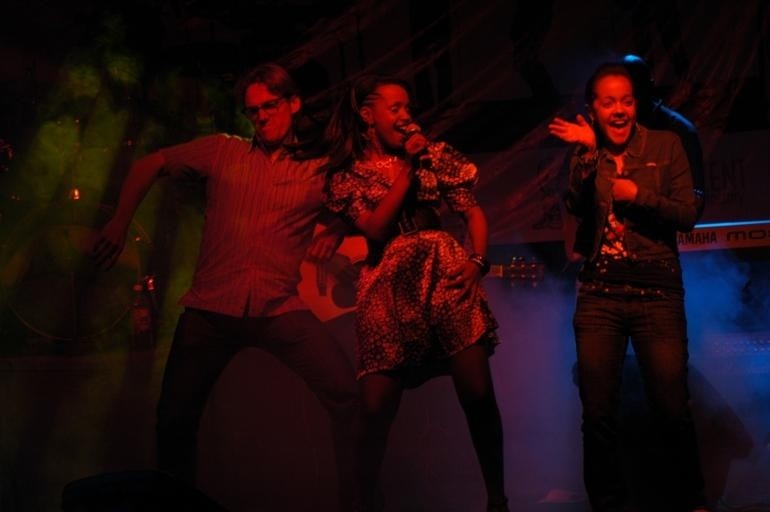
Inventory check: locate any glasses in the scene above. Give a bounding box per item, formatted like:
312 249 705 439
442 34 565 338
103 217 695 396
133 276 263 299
240 97 283 116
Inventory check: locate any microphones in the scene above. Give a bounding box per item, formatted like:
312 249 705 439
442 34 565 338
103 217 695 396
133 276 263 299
393 120 431 167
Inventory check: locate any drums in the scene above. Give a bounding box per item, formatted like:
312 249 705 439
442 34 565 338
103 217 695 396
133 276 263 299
6 196 149 344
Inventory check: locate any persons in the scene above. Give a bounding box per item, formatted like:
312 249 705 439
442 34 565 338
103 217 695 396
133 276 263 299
327 73 511 512
81 64 378 511
548 73 698 512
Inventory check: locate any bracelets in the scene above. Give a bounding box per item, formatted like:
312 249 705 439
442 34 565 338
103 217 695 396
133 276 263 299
469 254 490 277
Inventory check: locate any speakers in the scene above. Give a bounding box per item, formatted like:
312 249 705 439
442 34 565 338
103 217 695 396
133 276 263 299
573 354 752 512
